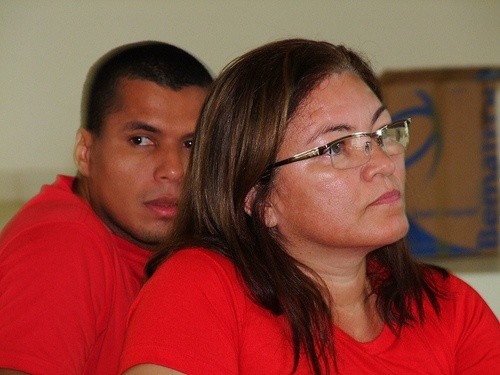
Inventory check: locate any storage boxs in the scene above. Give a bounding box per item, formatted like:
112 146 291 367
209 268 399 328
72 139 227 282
379 65 500 265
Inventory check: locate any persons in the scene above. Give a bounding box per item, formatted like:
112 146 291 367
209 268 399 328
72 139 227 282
109 36 500 375
0 40 217 375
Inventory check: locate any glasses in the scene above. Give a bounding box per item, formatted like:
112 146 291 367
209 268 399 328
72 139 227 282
257 117 411 171
